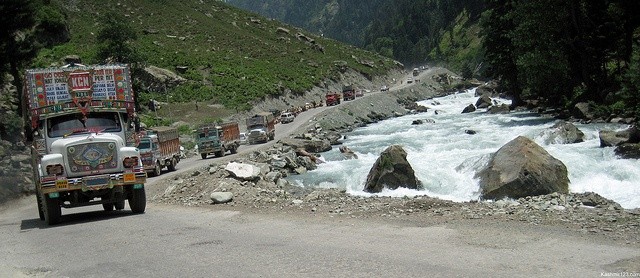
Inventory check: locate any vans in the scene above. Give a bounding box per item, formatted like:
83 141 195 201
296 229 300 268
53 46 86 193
281 112 294 124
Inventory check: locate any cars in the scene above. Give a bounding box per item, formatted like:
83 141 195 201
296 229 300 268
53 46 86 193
380 86 389 92
407 77 413 83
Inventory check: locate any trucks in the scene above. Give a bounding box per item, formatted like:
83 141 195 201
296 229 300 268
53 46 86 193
246 112 275 144
138 125 182 177
196 122 241 158
24 97 147 226
325 92 340 106
343 89 356 101
